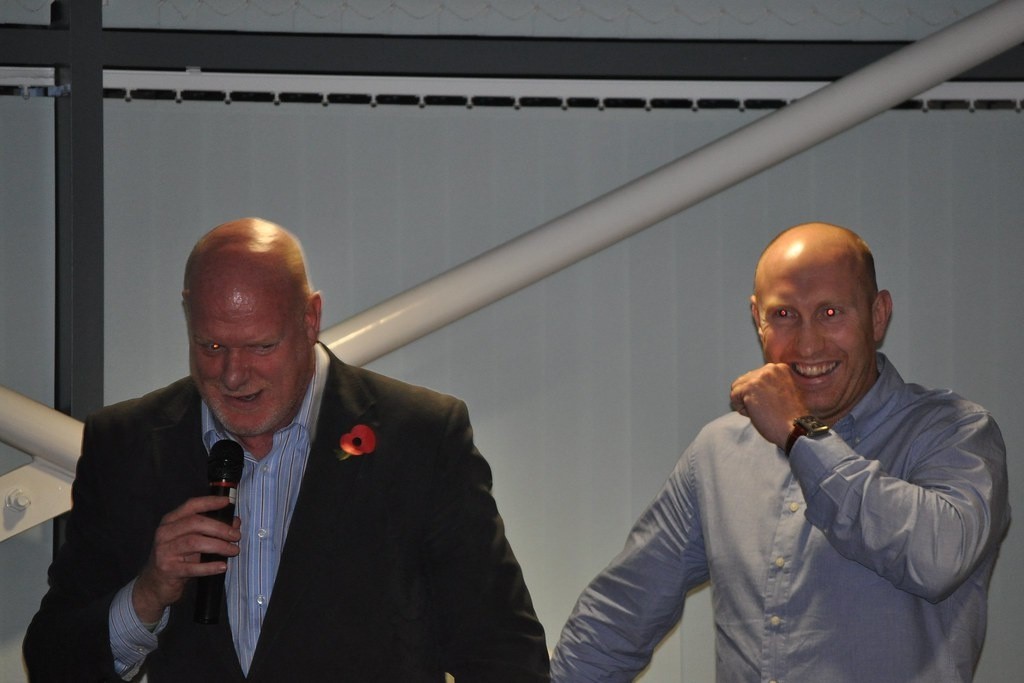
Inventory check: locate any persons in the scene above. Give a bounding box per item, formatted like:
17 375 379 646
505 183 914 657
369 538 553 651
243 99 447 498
22 217 551 683
549 222 1012 683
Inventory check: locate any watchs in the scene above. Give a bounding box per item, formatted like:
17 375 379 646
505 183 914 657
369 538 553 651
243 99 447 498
786 415 829 457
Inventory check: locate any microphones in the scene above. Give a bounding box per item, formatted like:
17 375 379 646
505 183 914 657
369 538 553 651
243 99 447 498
193 440 244 626
